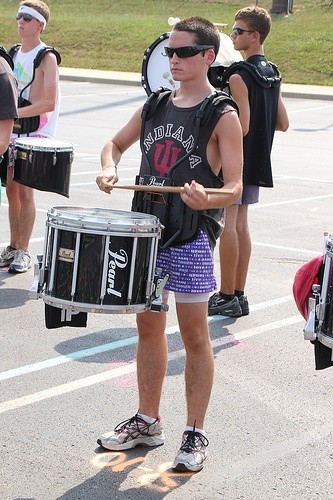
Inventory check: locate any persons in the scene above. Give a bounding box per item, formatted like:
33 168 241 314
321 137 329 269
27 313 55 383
0 43 22 156
209 6 289 320
96 16 244 474
0 0 63 273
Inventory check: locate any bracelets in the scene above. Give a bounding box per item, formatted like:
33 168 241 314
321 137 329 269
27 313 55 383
102 167 115 171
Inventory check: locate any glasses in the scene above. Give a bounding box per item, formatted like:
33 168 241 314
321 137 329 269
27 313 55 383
164 45 213 58
232 28 255 35
16 12 40 22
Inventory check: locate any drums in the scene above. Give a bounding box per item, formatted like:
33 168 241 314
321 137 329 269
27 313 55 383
30 206 170 315
141 33 245 97
303 232 333 353
13 136 74 199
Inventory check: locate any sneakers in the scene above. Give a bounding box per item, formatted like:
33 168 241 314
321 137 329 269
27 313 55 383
208 293 249 318
172 431 209 472
97 415 165 451
0 246 32 273
